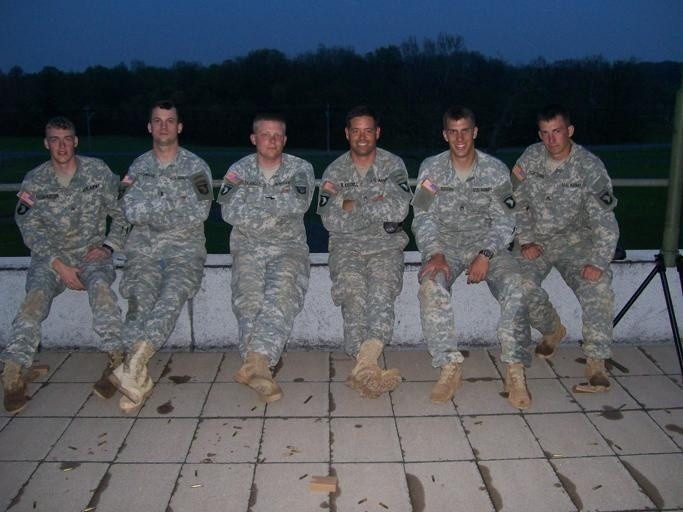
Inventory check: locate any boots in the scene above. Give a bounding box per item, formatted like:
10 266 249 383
93 369 112 397
236 351 282 403
587 354 609 391
364 369 404 397
2 365 25 414
348 339 386 399
534 324 569 361
507 364 530 409
118 367 154 412
106 340 144 407
433 365 461 403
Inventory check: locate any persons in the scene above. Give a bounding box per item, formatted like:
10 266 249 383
216 115 315 402
409 106 532 410
109 99 214 413
0 117 132 413
511 109 620 392
316 107 413 400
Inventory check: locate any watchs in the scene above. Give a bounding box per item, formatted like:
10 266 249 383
478 248 494 259
